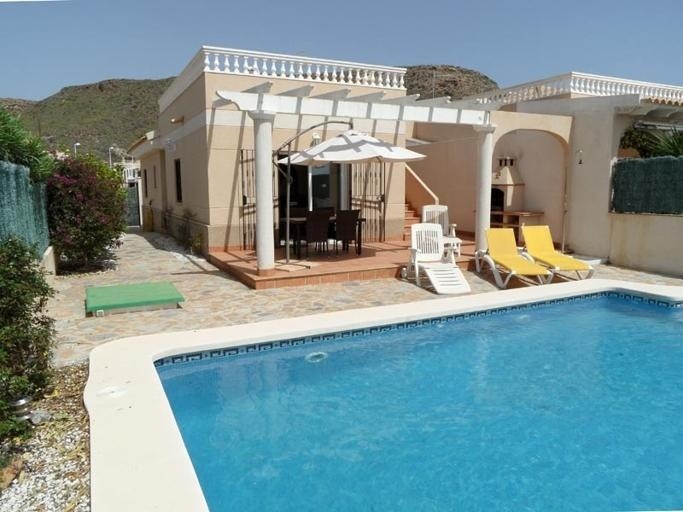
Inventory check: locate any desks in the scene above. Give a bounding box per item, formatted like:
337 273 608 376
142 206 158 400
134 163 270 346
282 217 366 259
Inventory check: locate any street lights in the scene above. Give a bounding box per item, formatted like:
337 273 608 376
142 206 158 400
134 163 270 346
74 143 81 159
109 147 114 169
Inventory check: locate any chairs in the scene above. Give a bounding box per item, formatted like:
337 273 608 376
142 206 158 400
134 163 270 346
307 206 361 256
404 205 594 296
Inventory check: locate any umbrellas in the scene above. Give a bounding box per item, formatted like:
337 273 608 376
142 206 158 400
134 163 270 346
277 128 428 211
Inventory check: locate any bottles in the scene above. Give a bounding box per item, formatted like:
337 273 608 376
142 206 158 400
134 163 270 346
402 268 407 281
